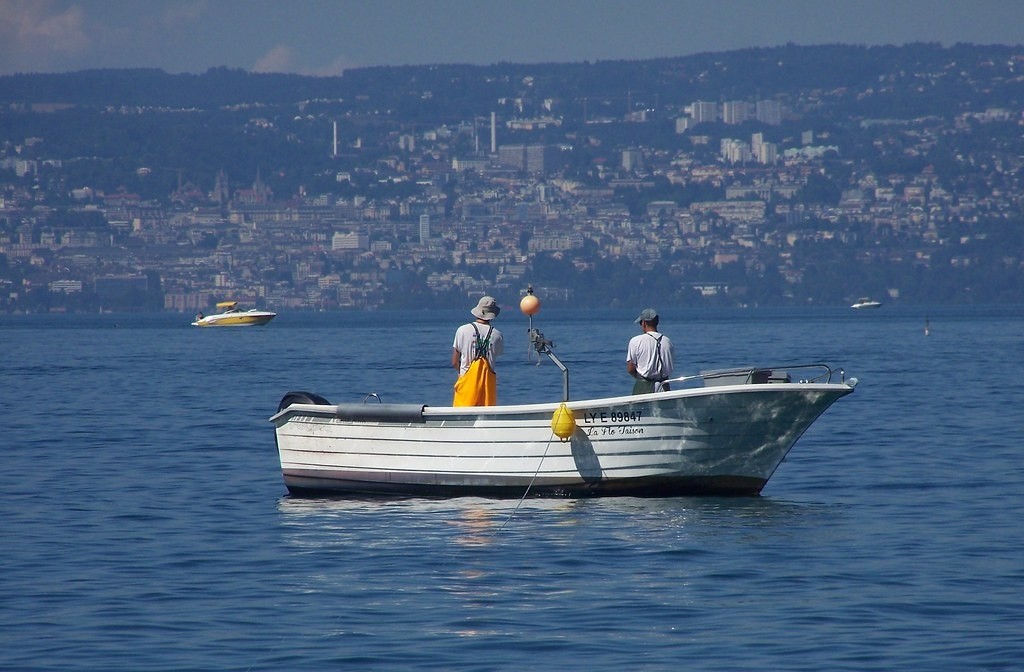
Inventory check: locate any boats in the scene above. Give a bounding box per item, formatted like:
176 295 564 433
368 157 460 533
270 285 862 498
190 301 278 326
851 296 884 309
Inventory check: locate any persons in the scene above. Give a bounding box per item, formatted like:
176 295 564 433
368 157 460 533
453 296 503 406
627 308 675 395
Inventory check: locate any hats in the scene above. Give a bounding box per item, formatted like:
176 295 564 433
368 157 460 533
634 309 658 323
471 296 499 320
